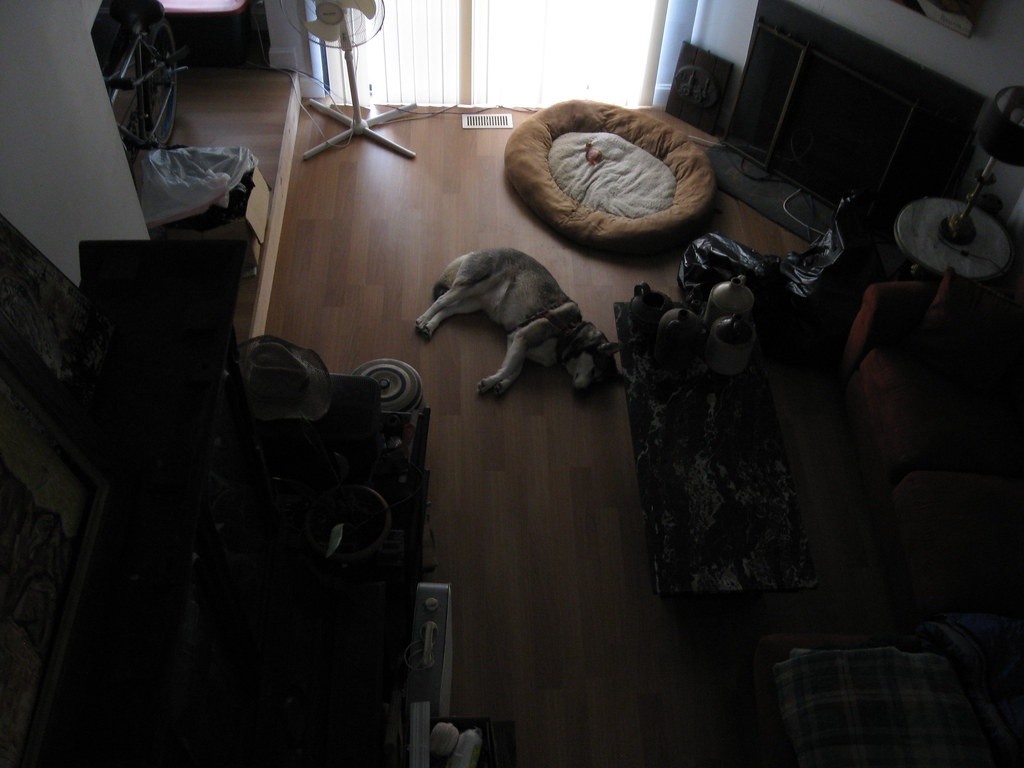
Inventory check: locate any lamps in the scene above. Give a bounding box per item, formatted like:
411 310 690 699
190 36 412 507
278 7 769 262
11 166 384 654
939 85 1024 246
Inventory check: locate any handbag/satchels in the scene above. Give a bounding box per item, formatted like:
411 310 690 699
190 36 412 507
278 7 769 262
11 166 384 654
677 232 785 314
779 245 829 298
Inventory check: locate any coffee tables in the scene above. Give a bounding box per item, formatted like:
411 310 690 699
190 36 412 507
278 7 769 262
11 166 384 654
613 302 823 598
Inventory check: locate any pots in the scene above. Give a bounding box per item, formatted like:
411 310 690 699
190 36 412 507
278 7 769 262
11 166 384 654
351 358 423 412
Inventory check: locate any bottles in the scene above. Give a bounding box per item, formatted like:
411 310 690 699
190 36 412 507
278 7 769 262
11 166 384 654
656 308 702 361
705 313 757 374
705 274 755 326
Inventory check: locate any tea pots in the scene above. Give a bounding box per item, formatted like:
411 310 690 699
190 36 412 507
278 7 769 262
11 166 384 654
629 284 674 334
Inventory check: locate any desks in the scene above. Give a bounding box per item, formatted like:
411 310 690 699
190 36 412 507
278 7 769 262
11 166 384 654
892 197 1015 281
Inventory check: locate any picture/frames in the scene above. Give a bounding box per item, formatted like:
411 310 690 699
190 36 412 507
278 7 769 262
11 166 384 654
0 309 145 768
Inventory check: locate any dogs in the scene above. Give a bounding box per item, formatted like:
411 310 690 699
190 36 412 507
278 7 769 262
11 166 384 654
412 245 627 403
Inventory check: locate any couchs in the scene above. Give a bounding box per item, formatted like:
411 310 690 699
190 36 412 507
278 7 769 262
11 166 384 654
733 270 1024 768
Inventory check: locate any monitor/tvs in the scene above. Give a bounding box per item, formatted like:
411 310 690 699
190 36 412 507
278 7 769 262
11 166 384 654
249 366 382 468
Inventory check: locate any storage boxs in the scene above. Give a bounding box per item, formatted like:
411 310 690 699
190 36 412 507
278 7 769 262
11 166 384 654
405 716 500 768
257 374 383 452
156 0 252 68
159 165 273 267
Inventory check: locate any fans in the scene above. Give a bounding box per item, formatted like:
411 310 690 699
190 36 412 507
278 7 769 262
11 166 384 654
280 0 419 160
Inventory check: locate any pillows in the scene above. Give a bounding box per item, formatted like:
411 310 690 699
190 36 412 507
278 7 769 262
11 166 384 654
903 264 1024 401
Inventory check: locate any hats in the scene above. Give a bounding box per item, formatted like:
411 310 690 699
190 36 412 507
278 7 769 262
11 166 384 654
235 334 333 422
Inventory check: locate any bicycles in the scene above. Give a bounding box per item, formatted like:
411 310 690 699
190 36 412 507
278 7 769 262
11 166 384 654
100 0 189 169
